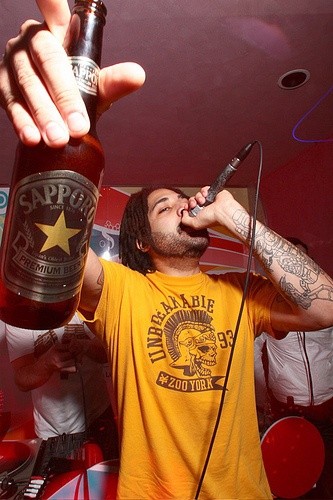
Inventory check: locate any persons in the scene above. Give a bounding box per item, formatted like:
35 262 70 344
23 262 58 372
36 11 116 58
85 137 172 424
4 309 108 440
0 0 333 500
253 237 333 500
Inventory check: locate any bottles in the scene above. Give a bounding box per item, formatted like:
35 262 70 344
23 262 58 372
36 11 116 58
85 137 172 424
0 0 107 330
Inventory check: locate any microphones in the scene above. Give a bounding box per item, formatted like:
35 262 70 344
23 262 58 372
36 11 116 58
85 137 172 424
188 140 256 217
